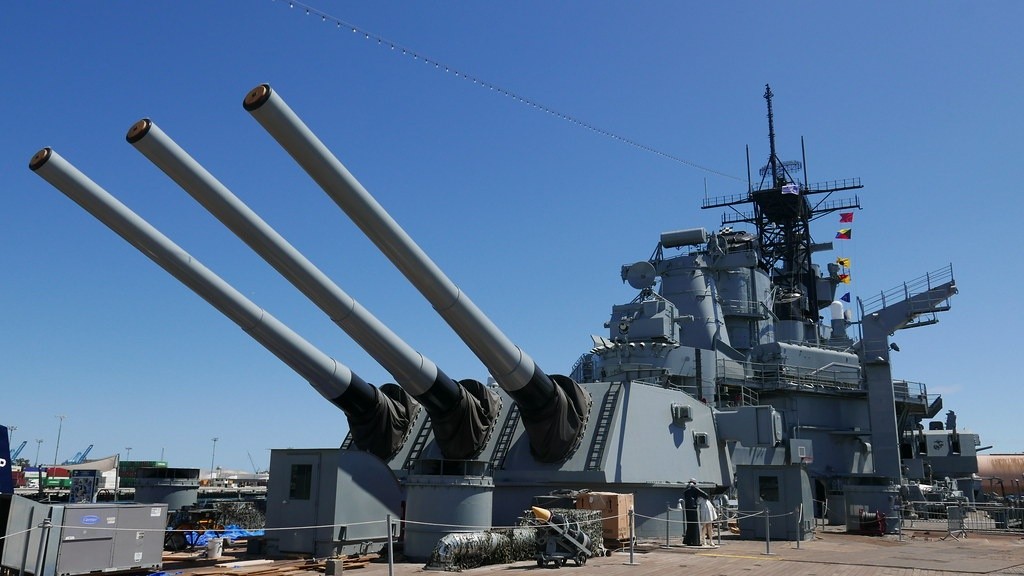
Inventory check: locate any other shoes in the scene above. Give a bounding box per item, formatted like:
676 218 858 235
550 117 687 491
702 540 707 545
709 541 714 546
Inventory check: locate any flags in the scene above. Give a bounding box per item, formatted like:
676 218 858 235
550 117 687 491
836 256 850 268
835 227 851 239
839 293 851 303
838 273 850 285
839 212 854 223
843 309 852 320
781 184 800 195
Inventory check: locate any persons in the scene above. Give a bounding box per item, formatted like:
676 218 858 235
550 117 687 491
681 478 718 546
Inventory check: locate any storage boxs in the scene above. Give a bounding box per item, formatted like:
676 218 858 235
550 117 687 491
577 492 634 539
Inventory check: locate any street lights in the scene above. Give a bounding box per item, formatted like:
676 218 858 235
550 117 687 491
211 438 219 471
7 424 19 443
54 414 69 466
126 447 132 461
35 439 45 467
